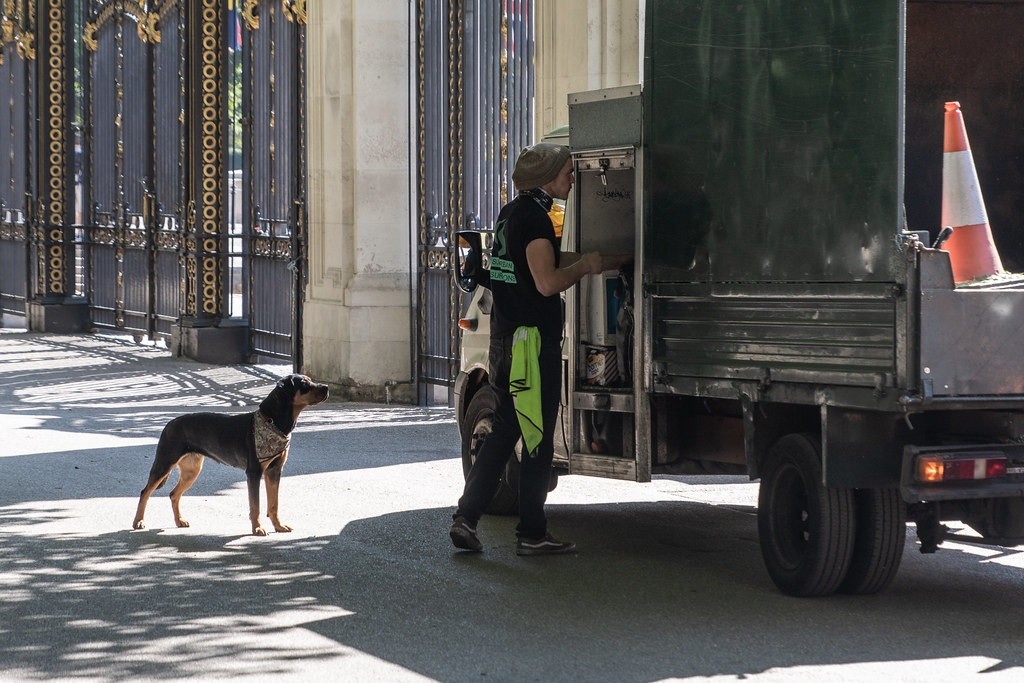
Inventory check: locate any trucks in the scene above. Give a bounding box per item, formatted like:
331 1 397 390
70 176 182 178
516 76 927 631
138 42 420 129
450 1 1023 599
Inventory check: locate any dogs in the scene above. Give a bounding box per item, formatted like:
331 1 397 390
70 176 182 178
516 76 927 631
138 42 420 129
132 374 330 538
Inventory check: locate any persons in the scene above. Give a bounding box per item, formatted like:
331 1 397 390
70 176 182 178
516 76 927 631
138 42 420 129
449 143 634 556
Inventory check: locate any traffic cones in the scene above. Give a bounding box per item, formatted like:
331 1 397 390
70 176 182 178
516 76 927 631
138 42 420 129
939 101 1024 290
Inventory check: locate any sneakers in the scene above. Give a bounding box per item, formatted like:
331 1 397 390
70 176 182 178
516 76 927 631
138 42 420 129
450 521 483 550
517 533 576 555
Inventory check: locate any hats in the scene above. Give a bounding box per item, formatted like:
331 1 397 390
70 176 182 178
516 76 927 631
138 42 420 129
512 142 570 192
